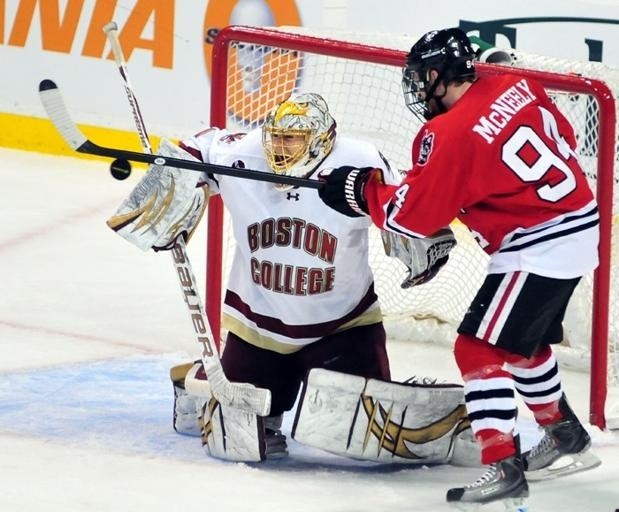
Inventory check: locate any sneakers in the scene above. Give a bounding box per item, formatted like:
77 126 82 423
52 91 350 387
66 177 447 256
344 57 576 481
523 419 591 471
447 455 529 504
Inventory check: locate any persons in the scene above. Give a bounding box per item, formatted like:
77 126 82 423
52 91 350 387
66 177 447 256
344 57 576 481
105 91 473 466
318 25 601 512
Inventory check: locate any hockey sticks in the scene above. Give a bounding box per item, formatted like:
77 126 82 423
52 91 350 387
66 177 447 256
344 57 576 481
39 79 326 188
103 21 271 416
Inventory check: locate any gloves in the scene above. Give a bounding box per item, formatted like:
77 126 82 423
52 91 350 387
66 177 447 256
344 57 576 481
318 167 385 217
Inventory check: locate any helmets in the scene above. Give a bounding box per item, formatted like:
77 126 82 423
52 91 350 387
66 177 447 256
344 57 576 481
402 28 476 124
261 94 337 192
